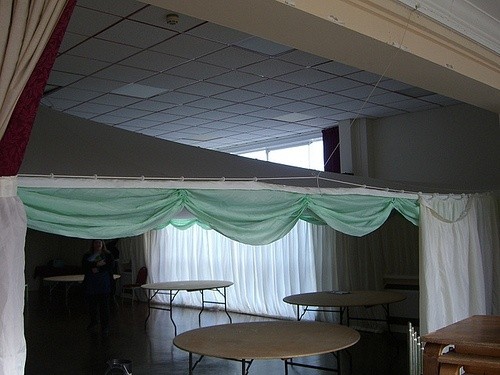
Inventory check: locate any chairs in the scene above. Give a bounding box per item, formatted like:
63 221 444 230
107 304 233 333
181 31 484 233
123 267 148 305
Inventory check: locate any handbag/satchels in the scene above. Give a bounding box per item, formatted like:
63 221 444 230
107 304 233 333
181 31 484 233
136 266 148 284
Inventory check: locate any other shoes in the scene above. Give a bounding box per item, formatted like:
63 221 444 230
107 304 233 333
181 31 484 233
87 320 98 328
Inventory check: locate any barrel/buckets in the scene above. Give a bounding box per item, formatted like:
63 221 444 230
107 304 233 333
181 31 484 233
106 358 133 375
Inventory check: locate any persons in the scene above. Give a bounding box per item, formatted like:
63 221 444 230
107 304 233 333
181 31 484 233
83 233 116 335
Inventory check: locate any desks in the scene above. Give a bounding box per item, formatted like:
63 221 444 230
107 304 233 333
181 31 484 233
420 315 500 375
43 274 121 314
283 290 407 351
173 320 363 375
141 280 234 338
